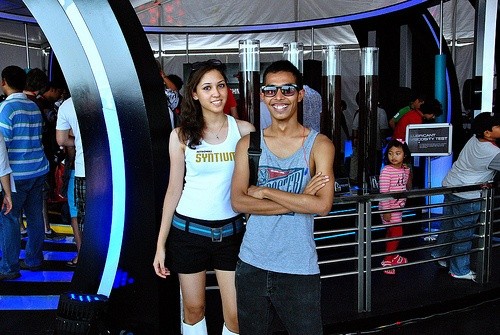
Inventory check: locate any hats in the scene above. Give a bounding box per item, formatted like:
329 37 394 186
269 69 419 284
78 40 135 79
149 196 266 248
474 112 500 127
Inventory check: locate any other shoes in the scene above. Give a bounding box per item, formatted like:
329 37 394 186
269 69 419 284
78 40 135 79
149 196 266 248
448 269 477 280
431 250 447 267
65 256 78 267
381 259 395 275
391 255 408 265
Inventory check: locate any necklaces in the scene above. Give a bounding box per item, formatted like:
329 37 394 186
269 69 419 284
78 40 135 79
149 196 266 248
215 116 226 140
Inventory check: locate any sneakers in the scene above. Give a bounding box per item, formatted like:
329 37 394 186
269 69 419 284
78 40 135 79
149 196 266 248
21 228 28 238
18 259 44 271
44 229 66 240
0 271 22 279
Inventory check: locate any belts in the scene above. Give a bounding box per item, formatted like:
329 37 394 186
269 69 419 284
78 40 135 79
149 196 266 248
173 215 247 242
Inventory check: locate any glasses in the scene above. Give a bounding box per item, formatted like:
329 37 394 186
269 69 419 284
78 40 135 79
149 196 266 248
190 58 222 77
262 84 297 97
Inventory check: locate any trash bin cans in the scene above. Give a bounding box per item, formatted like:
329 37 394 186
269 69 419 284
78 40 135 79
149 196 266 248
422 152 453 240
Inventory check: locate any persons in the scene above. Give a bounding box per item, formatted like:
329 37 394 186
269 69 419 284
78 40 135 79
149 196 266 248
352 106 389 195
260 84 272 130
303 79 323 133
391 98 443 141
431 112 500 279
156 59 183 130
379 138 413 275
230 60 335 335
0 66 86 280
153 58 256 335
389 98 424 129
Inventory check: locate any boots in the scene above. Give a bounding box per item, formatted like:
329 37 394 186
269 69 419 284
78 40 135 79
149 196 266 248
222 321 240 335
182 316 209 335
